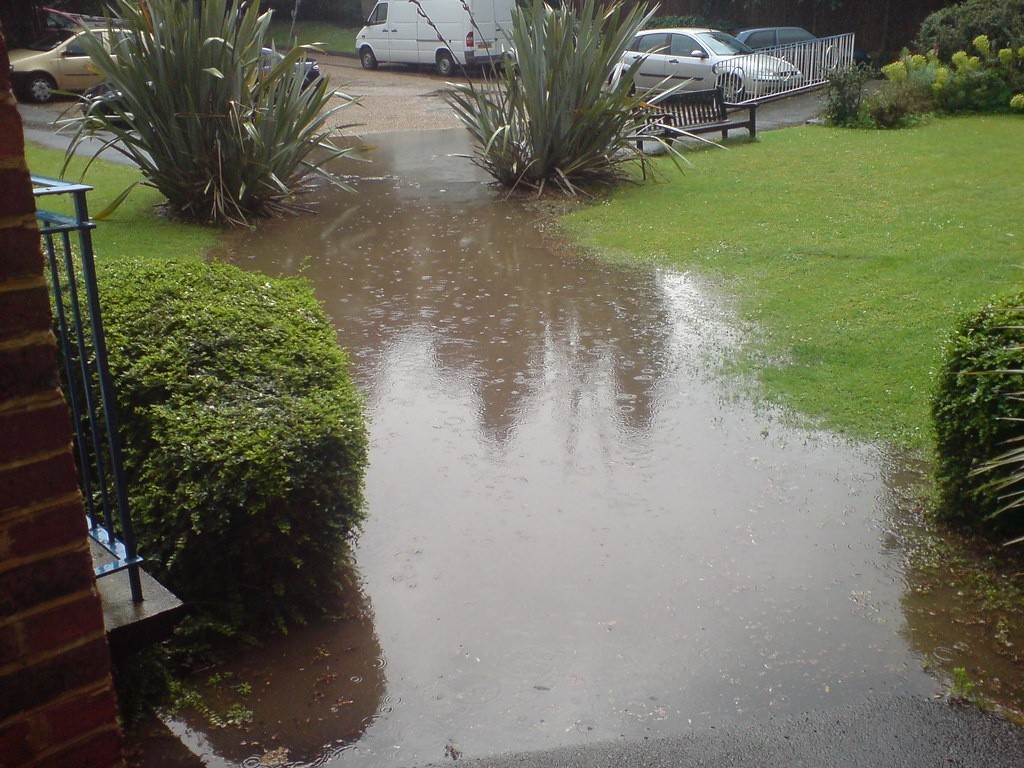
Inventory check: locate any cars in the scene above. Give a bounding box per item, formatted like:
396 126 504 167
606 27 803 102
728 27 846 77
48 0 159 37
35 7 88 36
7 28 174 102
80 41 322 129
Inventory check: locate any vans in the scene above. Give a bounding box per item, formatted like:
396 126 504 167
356 0 523 76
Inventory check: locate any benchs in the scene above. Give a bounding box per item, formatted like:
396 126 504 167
630 83 760 154
